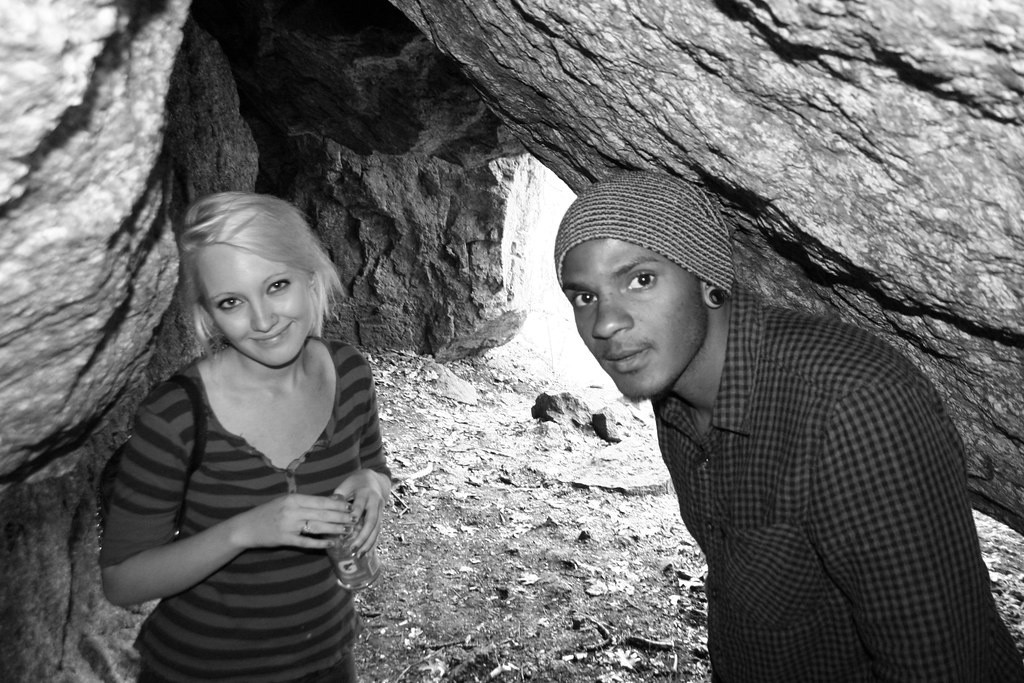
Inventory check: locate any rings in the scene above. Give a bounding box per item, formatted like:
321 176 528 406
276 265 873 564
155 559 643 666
306 519 309 533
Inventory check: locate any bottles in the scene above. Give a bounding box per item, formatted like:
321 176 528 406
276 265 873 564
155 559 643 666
326 495 381 589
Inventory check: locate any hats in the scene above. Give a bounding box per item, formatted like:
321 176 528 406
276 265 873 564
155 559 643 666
554 171 733 297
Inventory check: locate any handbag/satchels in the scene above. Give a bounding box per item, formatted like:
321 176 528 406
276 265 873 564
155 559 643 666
99 375 206 539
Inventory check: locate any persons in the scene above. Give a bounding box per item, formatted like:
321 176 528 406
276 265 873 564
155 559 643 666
100 191 392 683
554 171 1024 683
